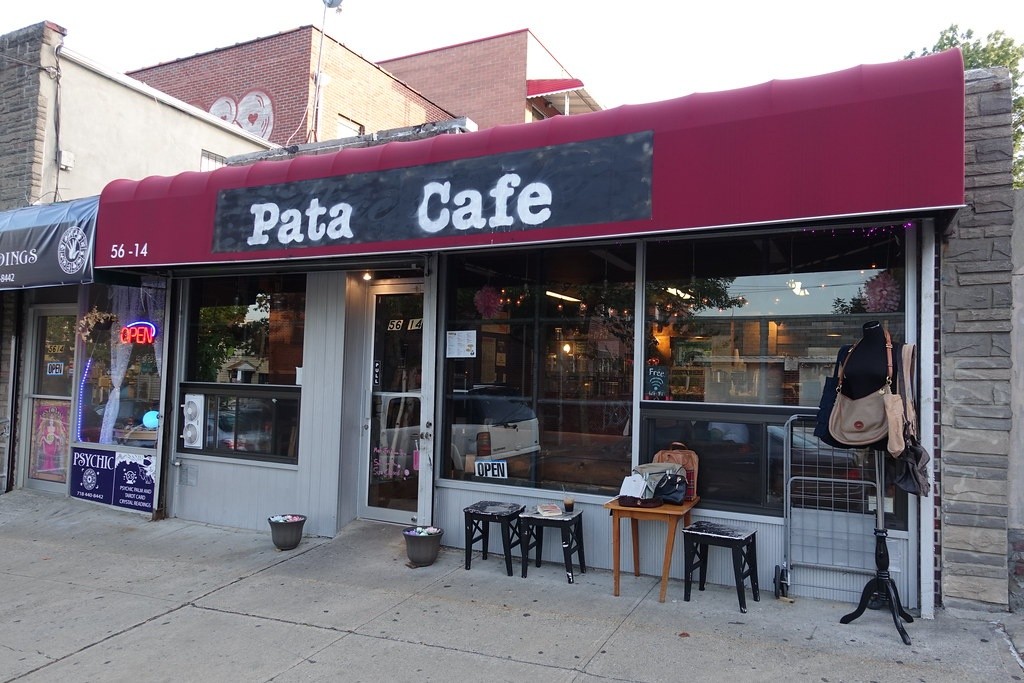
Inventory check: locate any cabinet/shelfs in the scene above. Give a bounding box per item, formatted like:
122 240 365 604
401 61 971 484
776 317 901 344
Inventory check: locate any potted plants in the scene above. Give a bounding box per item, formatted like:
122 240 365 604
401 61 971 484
77 306 120 343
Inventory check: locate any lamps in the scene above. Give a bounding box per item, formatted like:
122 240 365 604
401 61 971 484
681 239 709 292
545 101 552 108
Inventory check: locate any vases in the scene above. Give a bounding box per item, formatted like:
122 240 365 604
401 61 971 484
268 514 307 549
402 526 444 566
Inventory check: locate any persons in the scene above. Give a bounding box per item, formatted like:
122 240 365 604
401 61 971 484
704 384 751 472
840 320 914 452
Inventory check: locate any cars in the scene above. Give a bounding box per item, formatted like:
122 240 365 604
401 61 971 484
223 400 271 433
94 398 152 426
652 424 871 515
372 389 541 480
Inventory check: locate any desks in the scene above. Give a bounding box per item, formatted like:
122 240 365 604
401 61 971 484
112 429 157 440
603 497 700 603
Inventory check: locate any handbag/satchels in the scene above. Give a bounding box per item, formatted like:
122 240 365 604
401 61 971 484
619 473 664 507
829 330 907 458
652 442 699 500
652 470 688 506
887 342 930 497
633 462 688 499
813 346 870 450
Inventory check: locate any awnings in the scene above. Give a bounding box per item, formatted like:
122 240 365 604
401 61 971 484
0 195 165 290
94 46 965 273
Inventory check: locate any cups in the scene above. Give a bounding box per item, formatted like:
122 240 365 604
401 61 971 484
564 499 575 514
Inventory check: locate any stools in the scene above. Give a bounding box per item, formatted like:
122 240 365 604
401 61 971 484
462 500 528 577
680 520 760 613
517 507 586 584
